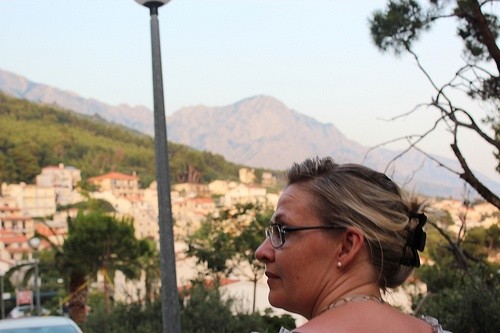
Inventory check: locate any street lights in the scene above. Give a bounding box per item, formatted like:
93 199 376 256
27 235 43 316
134 0 184 333
56 277 64 316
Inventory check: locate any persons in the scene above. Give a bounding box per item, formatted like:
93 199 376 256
255 154 453 333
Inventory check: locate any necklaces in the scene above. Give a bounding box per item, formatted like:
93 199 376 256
329 295 390 309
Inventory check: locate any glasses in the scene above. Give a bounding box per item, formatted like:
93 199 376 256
265 224 347 248
